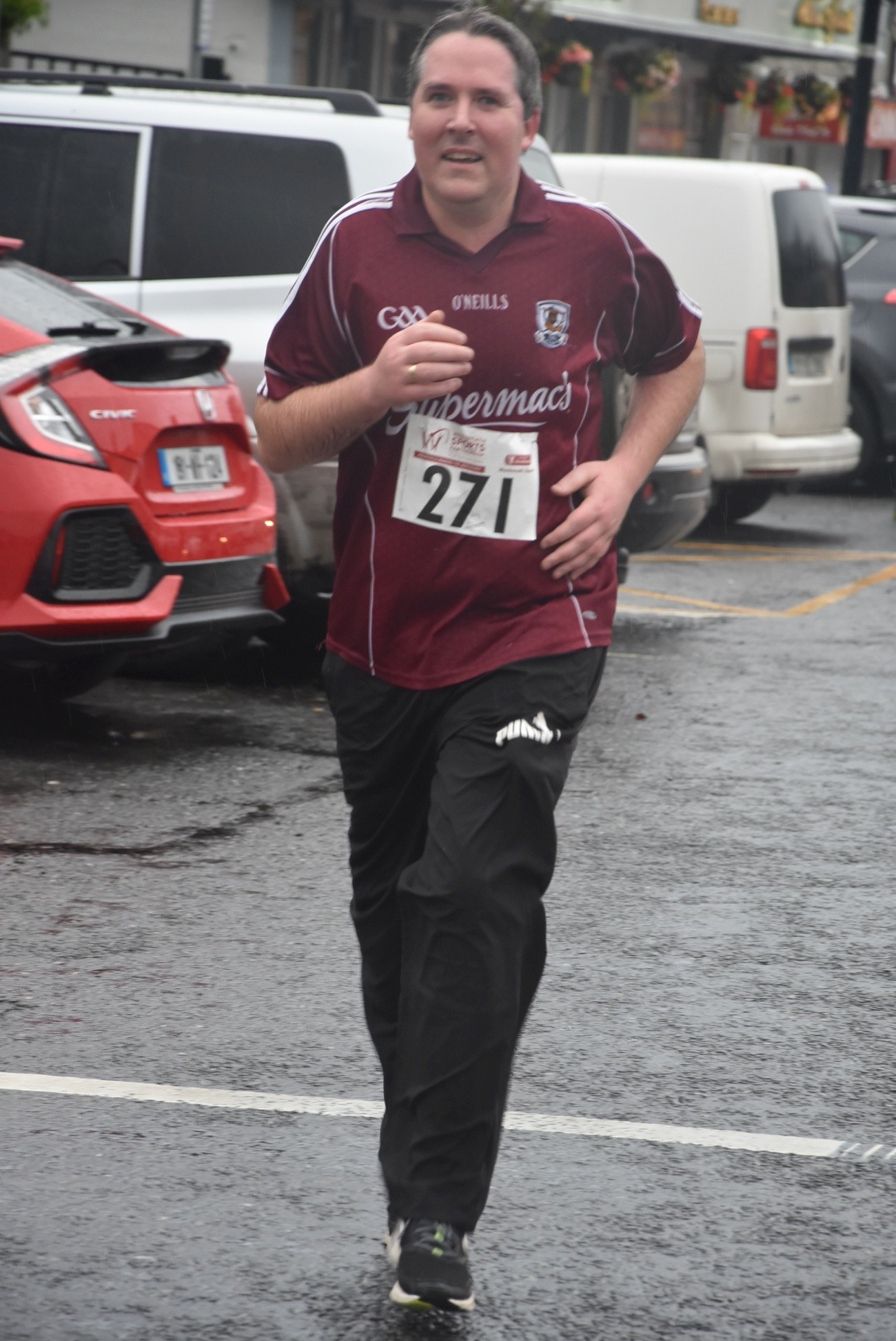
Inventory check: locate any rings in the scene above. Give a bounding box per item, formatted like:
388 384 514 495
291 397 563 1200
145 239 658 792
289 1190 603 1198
407 364 417 384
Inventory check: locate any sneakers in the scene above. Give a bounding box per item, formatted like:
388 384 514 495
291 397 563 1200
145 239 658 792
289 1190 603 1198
385 1214 478 1313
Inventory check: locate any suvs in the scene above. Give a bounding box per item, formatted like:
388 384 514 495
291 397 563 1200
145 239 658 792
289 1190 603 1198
780 192 896 497
1 65 565 654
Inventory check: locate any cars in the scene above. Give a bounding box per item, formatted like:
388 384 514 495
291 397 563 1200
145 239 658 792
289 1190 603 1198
597 364 715 586
0 235 291 701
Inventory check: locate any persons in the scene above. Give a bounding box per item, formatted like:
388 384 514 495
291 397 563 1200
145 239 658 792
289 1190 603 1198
252 9 706 1314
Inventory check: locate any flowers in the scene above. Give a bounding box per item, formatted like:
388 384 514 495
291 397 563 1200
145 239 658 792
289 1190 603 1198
608 44 683 99
710 58 857 122
538 31 596 99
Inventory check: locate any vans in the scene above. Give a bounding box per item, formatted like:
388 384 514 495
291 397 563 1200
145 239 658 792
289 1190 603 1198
555 149 864 541
